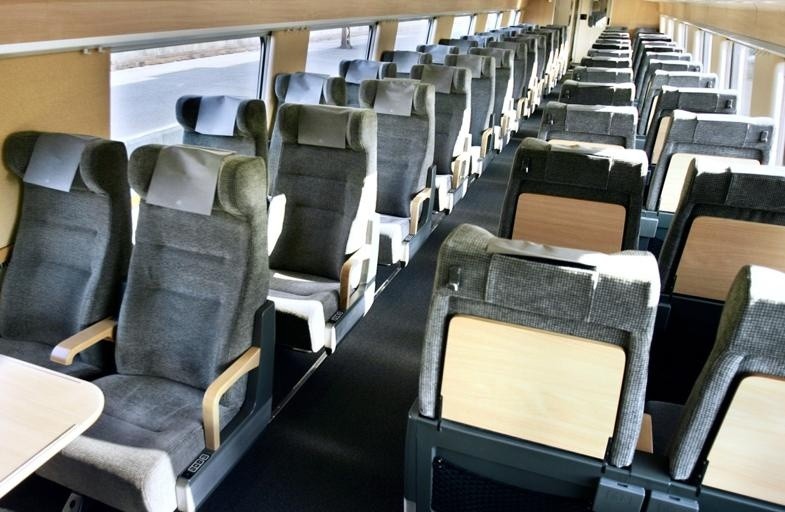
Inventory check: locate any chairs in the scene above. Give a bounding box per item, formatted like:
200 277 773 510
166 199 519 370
268 71 346 199
647 108 776 239
380 51 432 79
266 104 379 350
175 95 267 168
498 137 647 252
56 145 276 512
339 59 397 108
649 262 785 512
0 131 132 380
648 158 785 407
538 26 738 149
411 24 567 209
402 224 661 512
358 79 438 265
